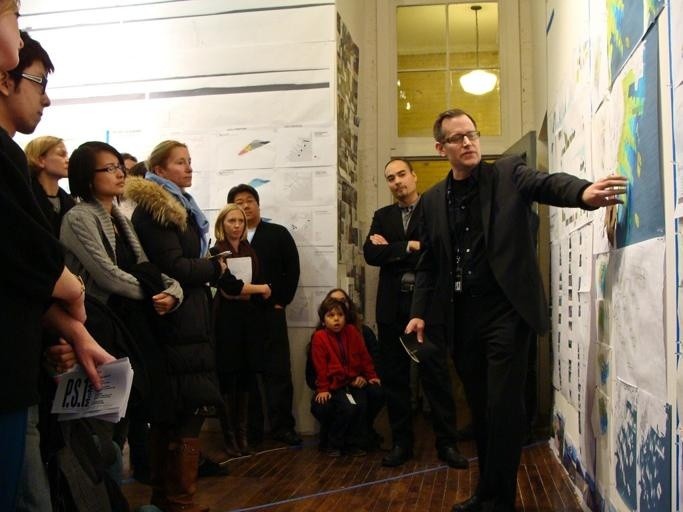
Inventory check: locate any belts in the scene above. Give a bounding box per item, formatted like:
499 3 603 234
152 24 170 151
401 282 415 293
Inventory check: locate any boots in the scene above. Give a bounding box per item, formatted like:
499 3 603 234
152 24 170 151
219 391 249 456
146 436 210 512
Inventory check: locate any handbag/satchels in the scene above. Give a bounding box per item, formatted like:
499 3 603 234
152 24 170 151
46 434 129 511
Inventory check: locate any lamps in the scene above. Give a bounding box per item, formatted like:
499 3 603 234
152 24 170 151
459 6 498 95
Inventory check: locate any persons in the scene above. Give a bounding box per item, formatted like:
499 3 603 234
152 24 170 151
58 139 299 510
404 108 629 512
309 298 382 456
456 335 538 448
304 289 386 453
0 0 117 512
363 159 470 469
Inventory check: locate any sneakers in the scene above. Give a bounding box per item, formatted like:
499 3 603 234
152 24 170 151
327 448 340 457
347 447 366 456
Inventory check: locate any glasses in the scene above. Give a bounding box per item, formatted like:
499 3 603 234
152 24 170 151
442 131 480 144
94 162 122 172
11 71 47 94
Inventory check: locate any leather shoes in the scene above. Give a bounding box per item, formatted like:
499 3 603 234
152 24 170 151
452 496 485 512
382 445 412 466
438 447 466 469
199 458 225 475
273 429 297 445
361 430 383 444
460 421 475 439
245 440 262 451
